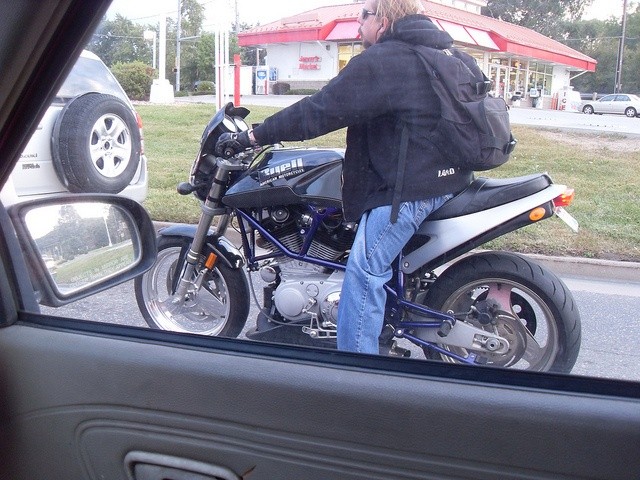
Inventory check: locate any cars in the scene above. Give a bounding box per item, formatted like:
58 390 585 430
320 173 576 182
578 94 640 118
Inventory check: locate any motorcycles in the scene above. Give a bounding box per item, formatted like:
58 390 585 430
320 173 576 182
133 100 582 379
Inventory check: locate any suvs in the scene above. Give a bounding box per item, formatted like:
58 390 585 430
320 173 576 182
1 48 150 206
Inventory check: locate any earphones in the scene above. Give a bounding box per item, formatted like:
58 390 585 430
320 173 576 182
376 21 386 34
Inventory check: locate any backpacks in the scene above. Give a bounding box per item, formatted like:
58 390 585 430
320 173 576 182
379 40 517 224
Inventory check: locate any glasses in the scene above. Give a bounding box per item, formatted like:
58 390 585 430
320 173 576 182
361 9 378 19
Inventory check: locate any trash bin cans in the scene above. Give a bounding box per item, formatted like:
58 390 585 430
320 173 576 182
512 96 520 107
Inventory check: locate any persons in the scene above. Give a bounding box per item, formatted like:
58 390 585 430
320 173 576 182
216 0 473 356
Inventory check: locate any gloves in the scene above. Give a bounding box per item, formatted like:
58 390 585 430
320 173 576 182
215 130 255 160
